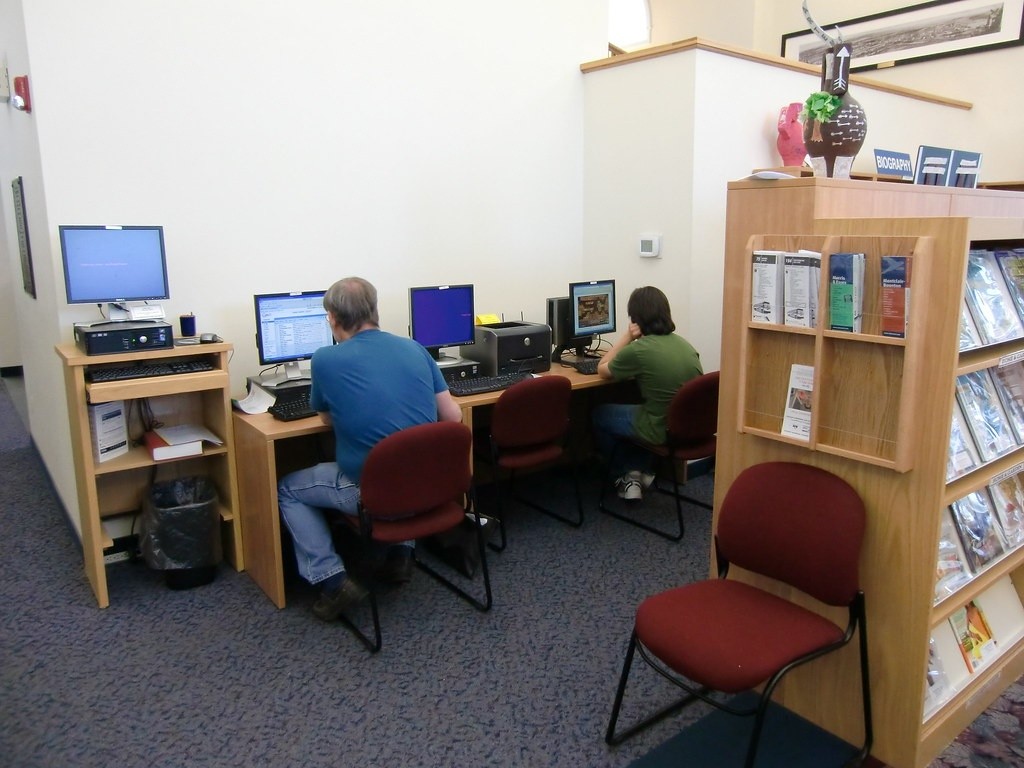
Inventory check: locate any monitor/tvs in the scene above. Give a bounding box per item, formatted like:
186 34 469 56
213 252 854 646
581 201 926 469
59 225 169 327
254 291 336 387
407 285 475 365
569 281 615 340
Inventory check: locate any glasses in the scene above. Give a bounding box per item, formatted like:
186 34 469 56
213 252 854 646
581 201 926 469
325 315 329 321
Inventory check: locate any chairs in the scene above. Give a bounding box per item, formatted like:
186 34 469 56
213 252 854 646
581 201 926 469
598 371 719 541
605 461 873 768
473 375 584 553
325 422 492 652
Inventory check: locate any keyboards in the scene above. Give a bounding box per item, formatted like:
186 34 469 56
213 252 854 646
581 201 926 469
267 398 318 421
86 359 214 384
573 359 600 375
446 373 525 397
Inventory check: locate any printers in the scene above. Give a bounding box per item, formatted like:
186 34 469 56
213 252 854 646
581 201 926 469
460 320 552 377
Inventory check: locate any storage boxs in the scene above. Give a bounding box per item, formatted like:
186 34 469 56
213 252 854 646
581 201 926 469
87 401 129 463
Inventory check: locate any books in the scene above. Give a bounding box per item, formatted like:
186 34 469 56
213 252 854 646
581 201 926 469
945 359 1024 484
923 597 998 720
959 248 1024 352
144 431 203 462
933 461 1024 607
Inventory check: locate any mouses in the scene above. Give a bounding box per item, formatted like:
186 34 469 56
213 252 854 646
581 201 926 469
199 333 217 342
520 372 534 379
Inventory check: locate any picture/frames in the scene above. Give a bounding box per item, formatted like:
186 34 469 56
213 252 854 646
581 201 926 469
781 0 1024 73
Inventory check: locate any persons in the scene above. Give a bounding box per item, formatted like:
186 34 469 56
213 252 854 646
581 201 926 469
592 286 703 499
277 277 462 620
965 600 994 658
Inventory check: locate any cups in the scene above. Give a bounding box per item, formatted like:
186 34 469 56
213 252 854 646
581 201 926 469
180 315 196 337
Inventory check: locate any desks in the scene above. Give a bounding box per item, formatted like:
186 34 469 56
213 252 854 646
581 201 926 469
231 346 636 609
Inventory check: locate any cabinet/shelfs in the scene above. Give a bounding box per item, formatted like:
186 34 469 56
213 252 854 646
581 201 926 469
54 332 244 609
709 177 1024 768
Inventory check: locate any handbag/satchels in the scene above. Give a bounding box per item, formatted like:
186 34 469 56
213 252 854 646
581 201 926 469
421 488 502 580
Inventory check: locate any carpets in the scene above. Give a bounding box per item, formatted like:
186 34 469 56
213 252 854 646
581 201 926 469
625 690 891 768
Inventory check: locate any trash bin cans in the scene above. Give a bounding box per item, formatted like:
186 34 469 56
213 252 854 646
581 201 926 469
137 475 221 591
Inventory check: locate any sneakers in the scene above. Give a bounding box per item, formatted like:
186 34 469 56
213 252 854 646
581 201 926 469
312 579 371 621
615 476 642 500
376 546 415 584
640 472 656 490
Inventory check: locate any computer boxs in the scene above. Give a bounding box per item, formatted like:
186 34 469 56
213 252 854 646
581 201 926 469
73 321 174 357
246 369 313 407
547 297 592 347
434 357 481 383
